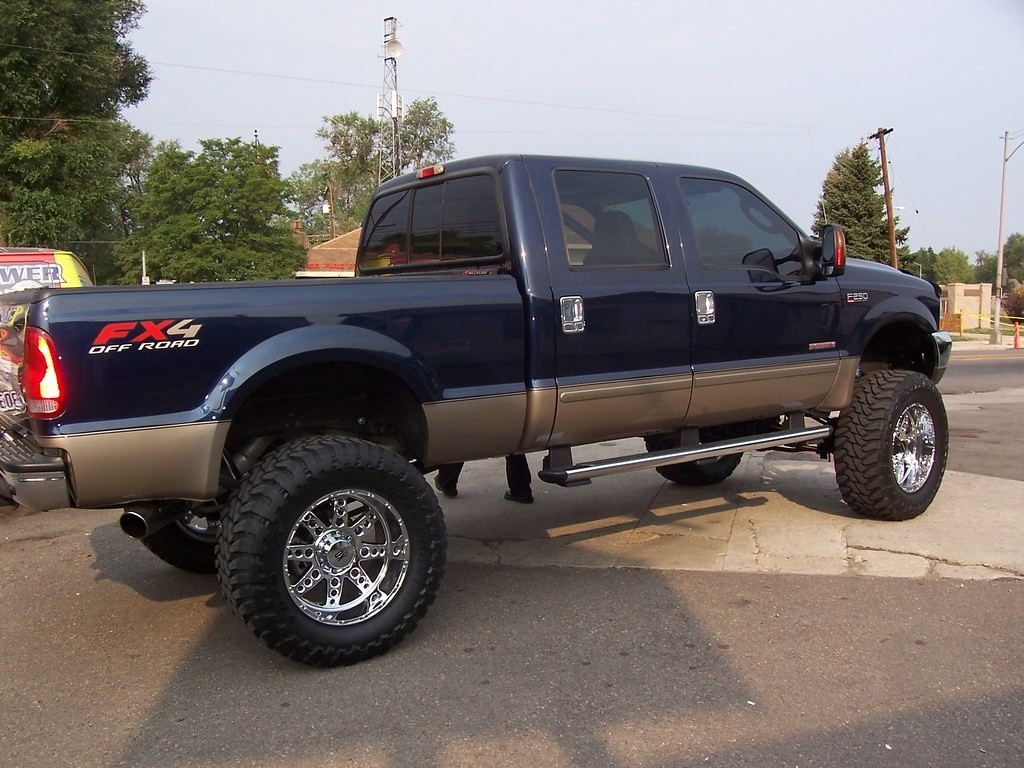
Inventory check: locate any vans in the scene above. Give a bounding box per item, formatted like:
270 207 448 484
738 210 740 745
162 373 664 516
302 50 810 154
0 247 96 410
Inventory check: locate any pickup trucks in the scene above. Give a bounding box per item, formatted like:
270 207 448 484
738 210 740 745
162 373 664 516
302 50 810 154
0 153 951 667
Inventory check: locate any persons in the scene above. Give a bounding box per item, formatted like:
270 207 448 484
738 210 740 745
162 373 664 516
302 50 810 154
434 453 534 504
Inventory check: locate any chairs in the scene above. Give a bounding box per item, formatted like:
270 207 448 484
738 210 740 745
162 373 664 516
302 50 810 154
583 210 664 267
465 182 506 259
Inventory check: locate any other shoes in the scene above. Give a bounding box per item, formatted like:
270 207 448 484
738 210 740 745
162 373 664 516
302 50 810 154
505 490 533 504
435 474 459 496
